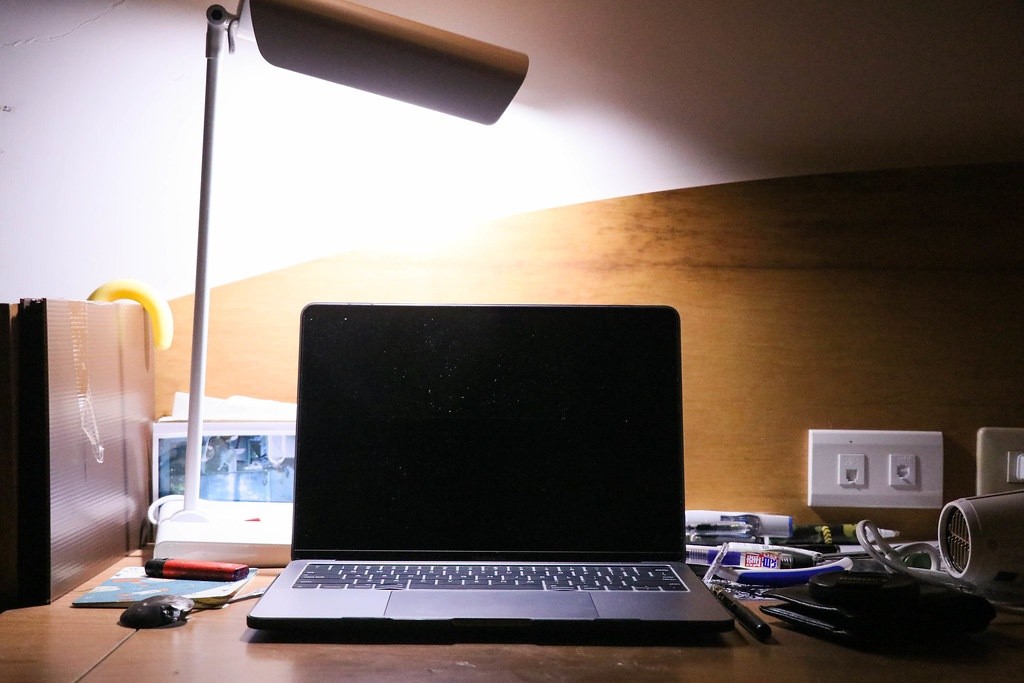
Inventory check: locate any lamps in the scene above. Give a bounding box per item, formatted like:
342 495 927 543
146 0 529 566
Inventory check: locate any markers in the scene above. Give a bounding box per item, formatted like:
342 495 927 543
686 549 817 570
710 584 772 643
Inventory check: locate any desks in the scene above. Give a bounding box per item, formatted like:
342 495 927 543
1 541 1024 682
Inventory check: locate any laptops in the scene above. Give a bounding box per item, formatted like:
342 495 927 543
248 302 732 636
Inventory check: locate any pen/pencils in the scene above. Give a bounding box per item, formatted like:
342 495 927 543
685 521 754 536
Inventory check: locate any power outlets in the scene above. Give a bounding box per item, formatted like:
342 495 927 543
976 427 1023 497
